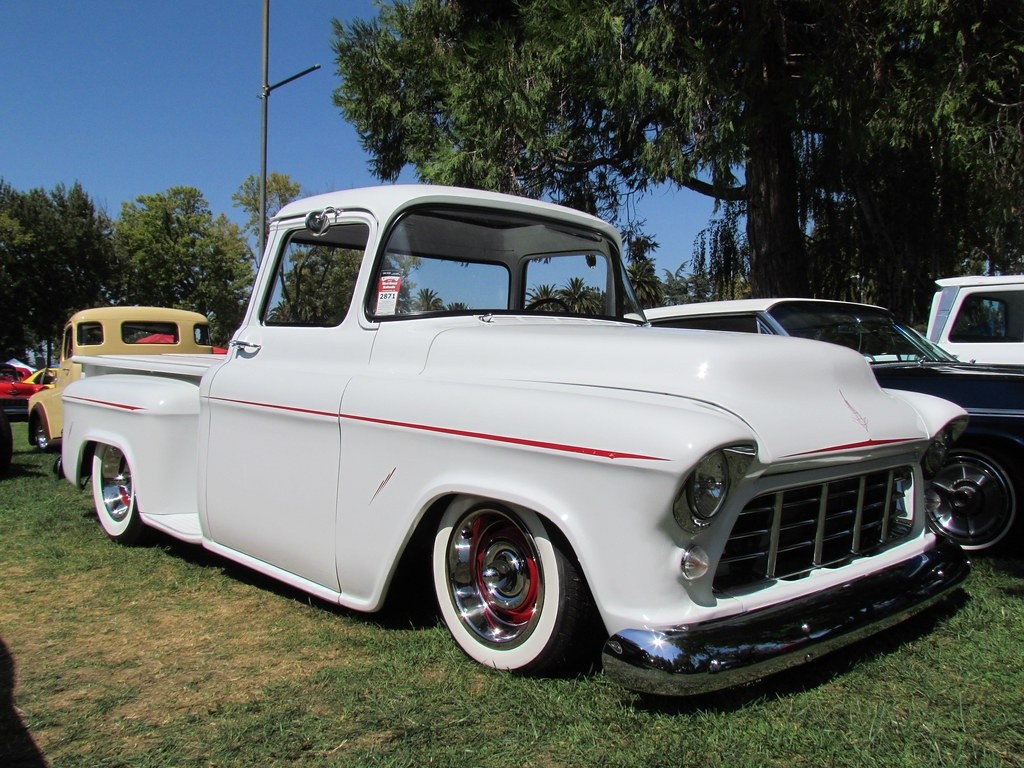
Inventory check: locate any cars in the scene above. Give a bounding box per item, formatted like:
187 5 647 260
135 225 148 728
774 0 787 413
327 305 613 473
26 306 214 479
57 183 976 702
924 272 1024 368
0 363 50 422
21 368 59 390
619 296 1023 554
